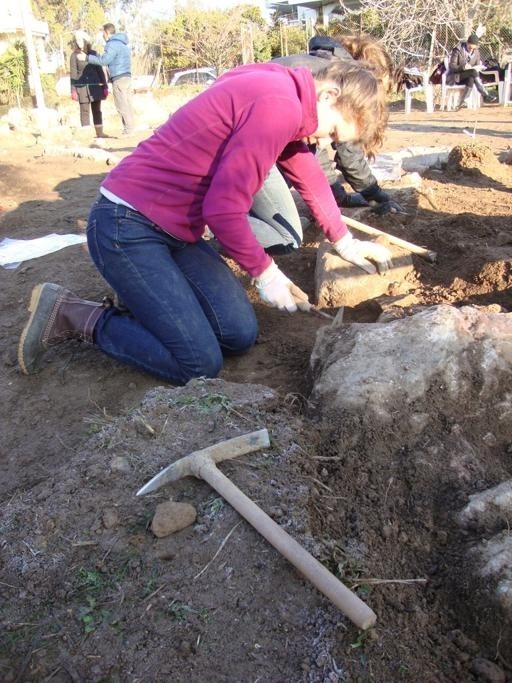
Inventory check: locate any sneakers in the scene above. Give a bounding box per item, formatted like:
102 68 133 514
120 125 133 134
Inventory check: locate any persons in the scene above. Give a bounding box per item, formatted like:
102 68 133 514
67 28 111 139
208 30 406 260
17 58 393 388
446 34 496 109
74 23 138 139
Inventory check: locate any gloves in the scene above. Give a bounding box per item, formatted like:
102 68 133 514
103 88 108 97
361 184 393 205
331 231 394 277
70 90 79 101
255 262 309 315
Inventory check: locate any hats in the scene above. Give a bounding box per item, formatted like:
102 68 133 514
468 35 480 46
308 36 341 52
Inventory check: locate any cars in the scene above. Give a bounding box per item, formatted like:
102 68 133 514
170 67 226 86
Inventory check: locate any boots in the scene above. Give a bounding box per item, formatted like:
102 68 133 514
16 281 108 375
474 77 497 103
457 86 474 111
94 124 110 138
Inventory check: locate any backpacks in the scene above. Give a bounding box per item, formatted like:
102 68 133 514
331 181 371 207
